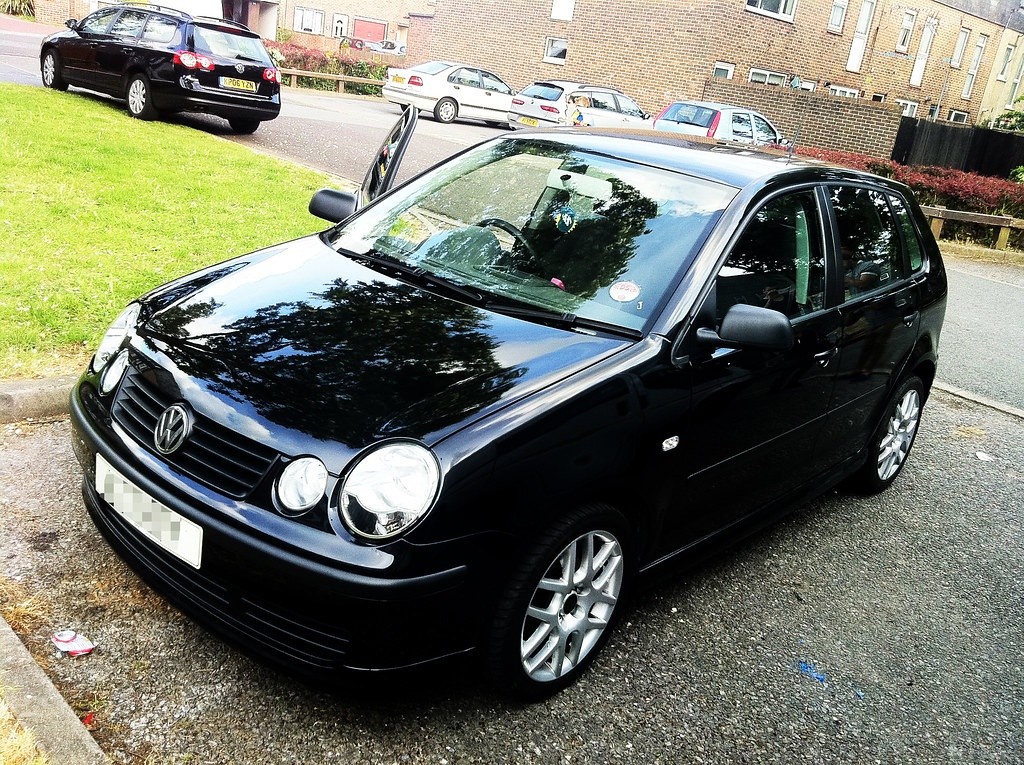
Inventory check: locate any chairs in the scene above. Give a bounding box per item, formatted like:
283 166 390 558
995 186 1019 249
522 203 613 291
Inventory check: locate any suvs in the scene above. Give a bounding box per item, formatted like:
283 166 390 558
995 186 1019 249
39 2 282 135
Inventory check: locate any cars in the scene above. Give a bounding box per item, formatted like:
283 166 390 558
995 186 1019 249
382 59 517 127
68 104 949 699
334 34 405 55
507 78 654 132
652 99 790 149
